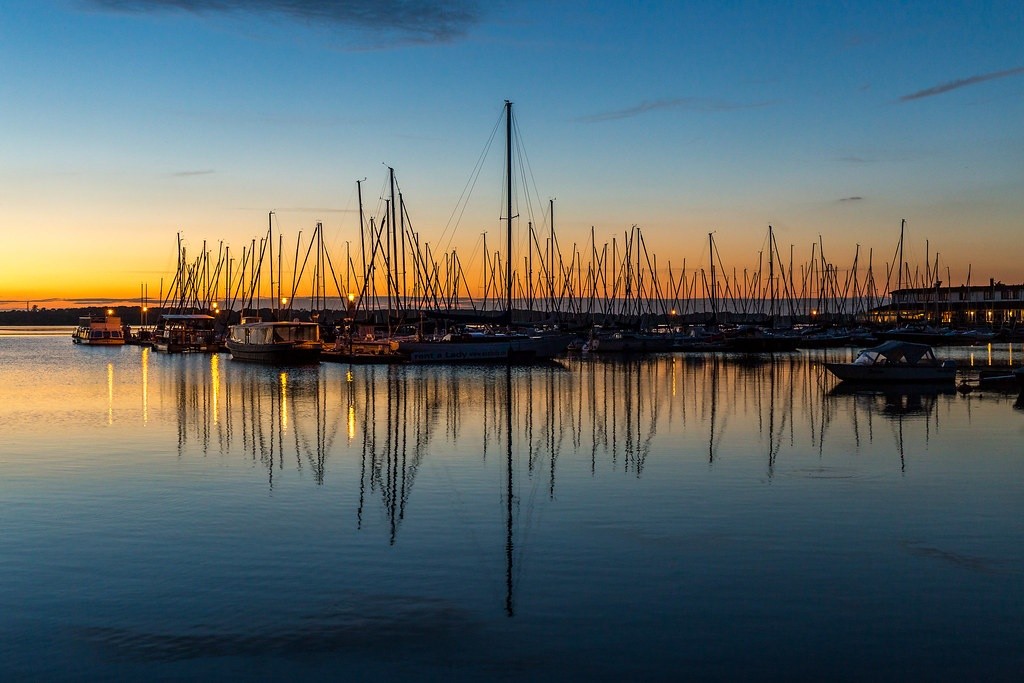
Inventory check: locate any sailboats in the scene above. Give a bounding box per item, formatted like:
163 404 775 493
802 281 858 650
71 100 1014 363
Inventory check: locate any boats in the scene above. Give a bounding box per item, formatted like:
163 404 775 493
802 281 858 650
819 339 959 387
